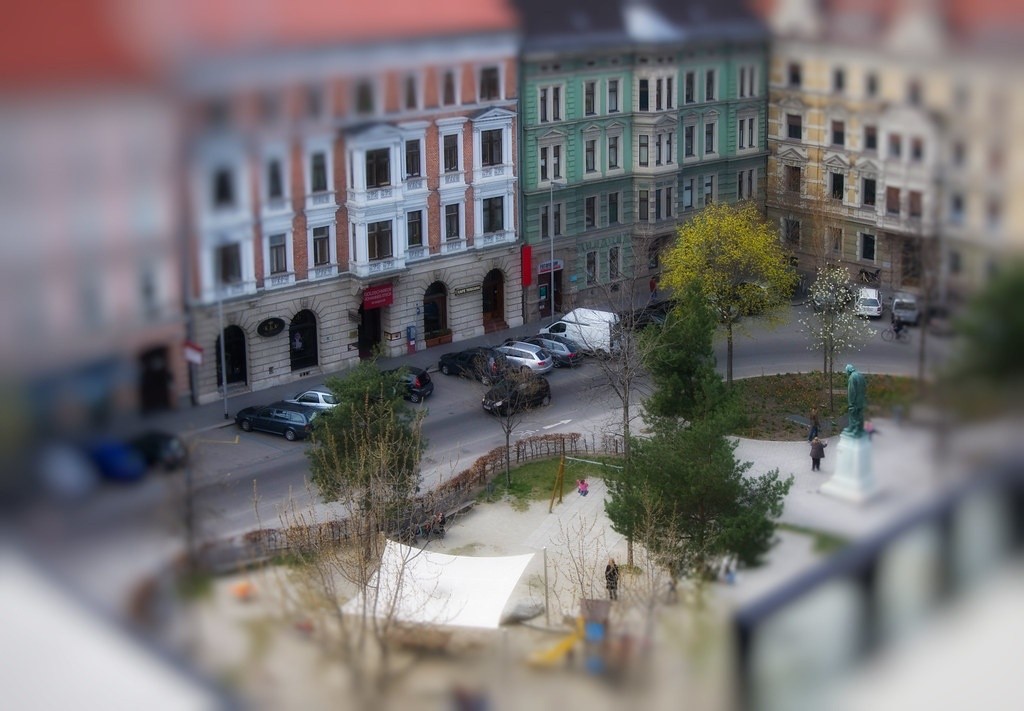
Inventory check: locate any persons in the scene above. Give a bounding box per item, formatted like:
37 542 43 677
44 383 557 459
605 558 620 599
844 364 866 433
576 479 589 496
808 407 819 442
810 437 829 471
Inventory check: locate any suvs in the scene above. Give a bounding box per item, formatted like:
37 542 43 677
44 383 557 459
506 332 585 368
234 400 322 441
490 339 554 381
888 292 919 327
438 345 513 385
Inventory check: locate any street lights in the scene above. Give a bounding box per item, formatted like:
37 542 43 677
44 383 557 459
540 175 569 323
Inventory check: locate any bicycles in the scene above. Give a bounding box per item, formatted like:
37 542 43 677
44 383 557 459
881 321 913 345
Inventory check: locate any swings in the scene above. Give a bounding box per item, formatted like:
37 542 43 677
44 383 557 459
574 460 591 497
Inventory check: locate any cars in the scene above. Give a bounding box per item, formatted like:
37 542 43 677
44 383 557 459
283 384 343 414
379 363 436 402
812 283 852 315
31 426 191 498
854 287 884 319
616 274 784 345
482 372 551 417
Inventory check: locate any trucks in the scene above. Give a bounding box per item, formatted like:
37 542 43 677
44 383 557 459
535 307 623 361
